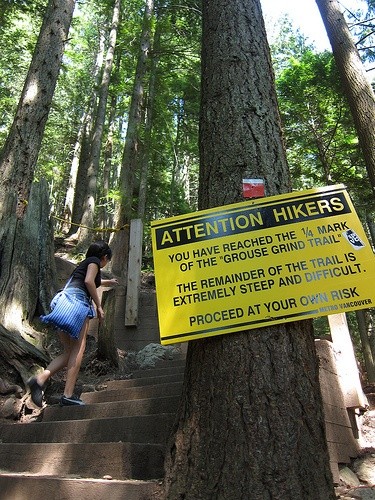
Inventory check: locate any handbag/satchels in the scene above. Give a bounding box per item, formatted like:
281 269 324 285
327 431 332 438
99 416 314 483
44 291 90 337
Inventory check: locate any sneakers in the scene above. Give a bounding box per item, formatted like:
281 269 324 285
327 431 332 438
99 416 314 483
60 394 85 406
26 376 43 408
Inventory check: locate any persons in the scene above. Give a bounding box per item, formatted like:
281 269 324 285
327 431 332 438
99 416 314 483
27 243 119 407
